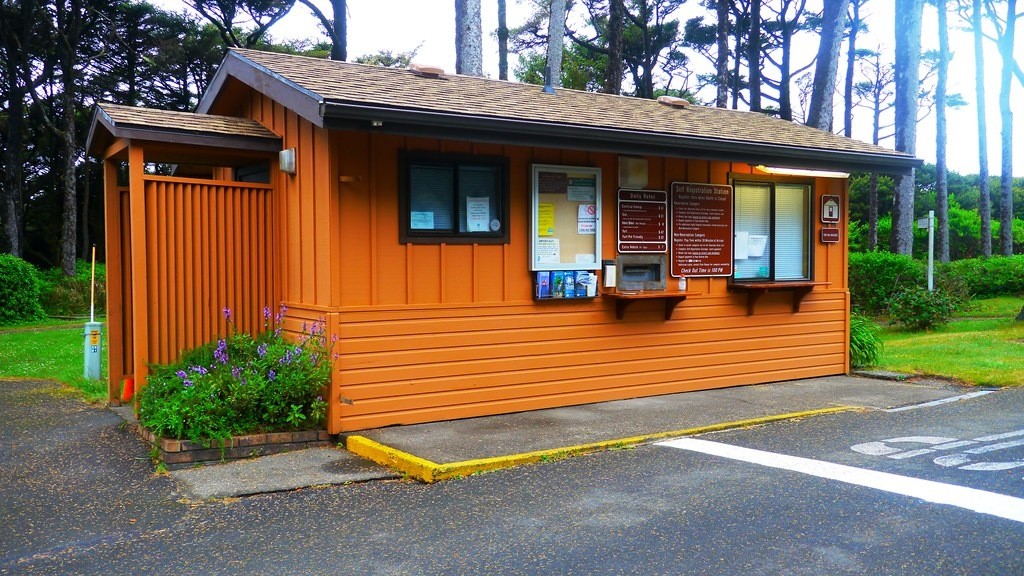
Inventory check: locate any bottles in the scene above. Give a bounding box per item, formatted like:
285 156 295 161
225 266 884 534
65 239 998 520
679 274 685 292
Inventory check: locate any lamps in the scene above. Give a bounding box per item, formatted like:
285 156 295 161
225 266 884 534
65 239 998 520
278 147 296 175
756 165 850 179
